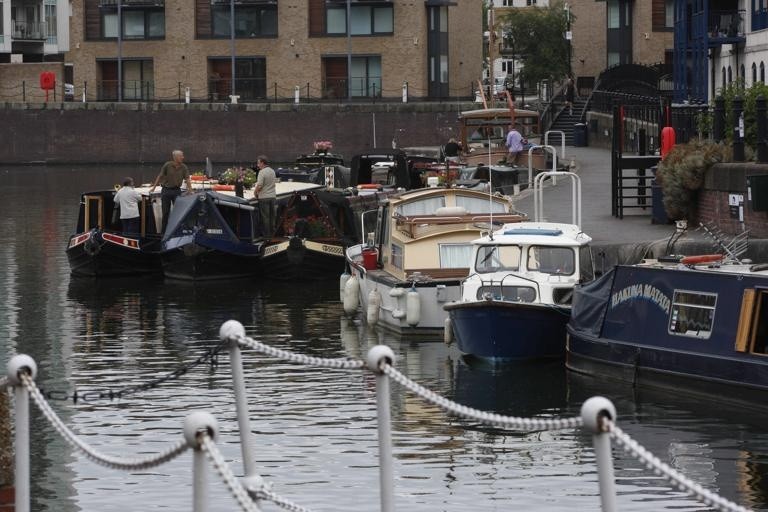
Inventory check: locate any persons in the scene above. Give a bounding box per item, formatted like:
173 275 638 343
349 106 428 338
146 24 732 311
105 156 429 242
443 137 462 163
113 175 145 237
148 149 195 238
564 78 581 118
502 122 529 166
253 155 277 241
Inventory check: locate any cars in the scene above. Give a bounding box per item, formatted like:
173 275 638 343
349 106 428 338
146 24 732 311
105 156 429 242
64 83 74 101
473 77 514 101
